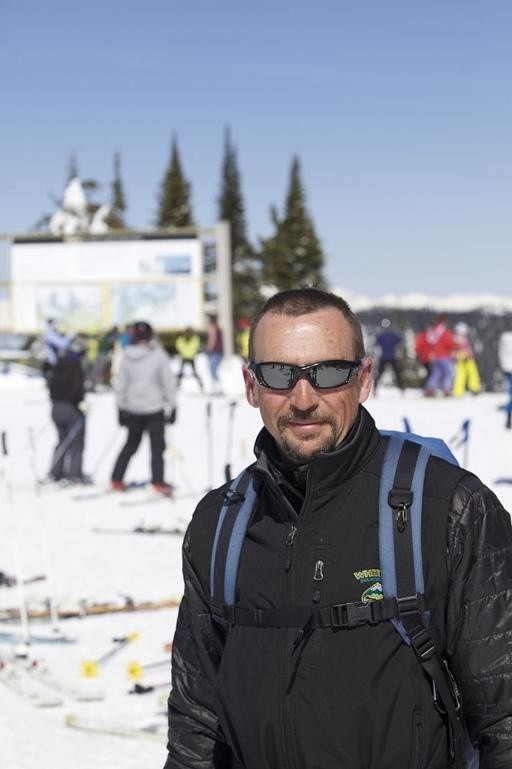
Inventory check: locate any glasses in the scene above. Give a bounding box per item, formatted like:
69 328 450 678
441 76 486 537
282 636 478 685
248 360 361 390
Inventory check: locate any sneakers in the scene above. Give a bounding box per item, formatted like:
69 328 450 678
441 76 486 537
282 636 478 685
51 472 127 491
423 388 483 396
153 482 173 495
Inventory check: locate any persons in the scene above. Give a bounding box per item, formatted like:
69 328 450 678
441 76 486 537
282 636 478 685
366 311 512 427
36 308 252 497
162 289 512 769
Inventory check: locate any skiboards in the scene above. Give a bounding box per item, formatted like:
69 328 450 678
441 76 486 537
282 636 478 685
0 581 179 741
69 481 211 505
